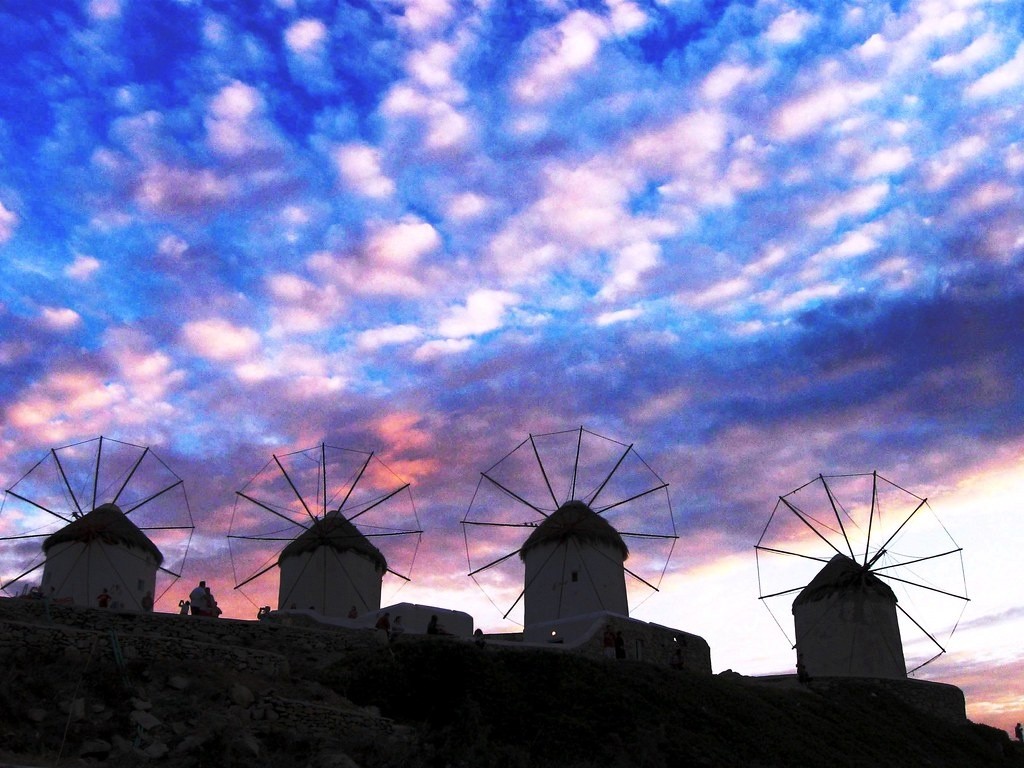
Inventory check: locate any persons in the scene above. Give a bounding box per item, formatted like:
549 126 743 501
257 606 274 623
348 605 359 619
1015 723 1024 742
796 653 811 684
290 601 297 609
97 589 112 608
42 587 57 600
427 614 441 635
375 612 391 633
309 606 315 610
141 590 154 612
392 615 405 631
603 625 627 660
179 581 222 618
